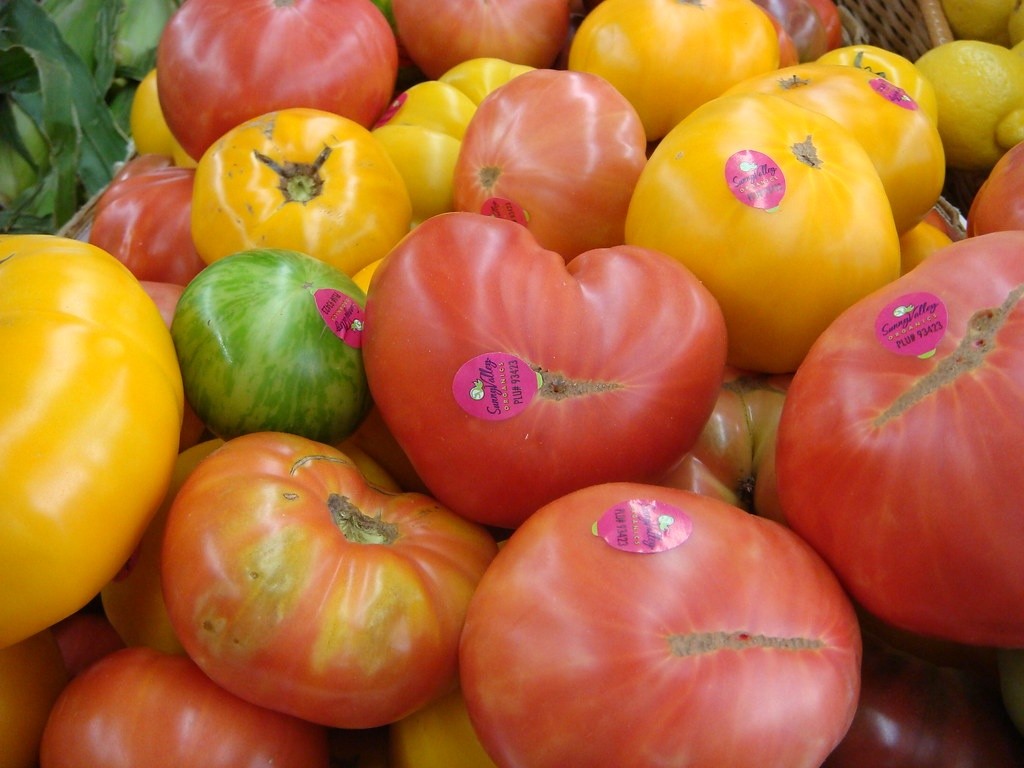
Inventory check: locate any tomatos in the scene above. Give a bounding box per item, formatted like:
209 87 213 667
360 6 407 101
0 0 1024 768
